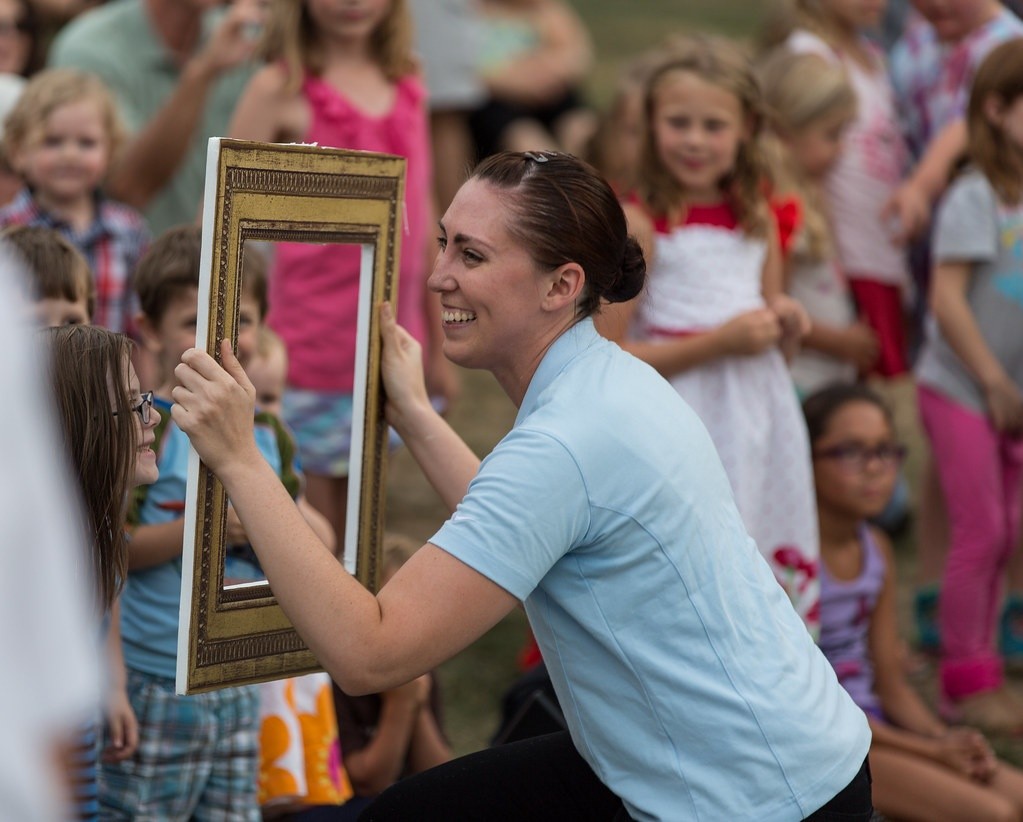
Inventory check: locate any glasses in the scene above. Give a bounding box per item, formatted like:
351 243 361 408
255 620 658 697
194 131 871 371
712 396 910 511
92 390 152 424
813 440 907 465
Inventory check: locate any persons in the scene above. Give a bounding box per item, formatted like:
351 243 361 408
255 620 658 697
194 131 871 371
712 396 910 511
1 1 1022 822
170 149 875 822
